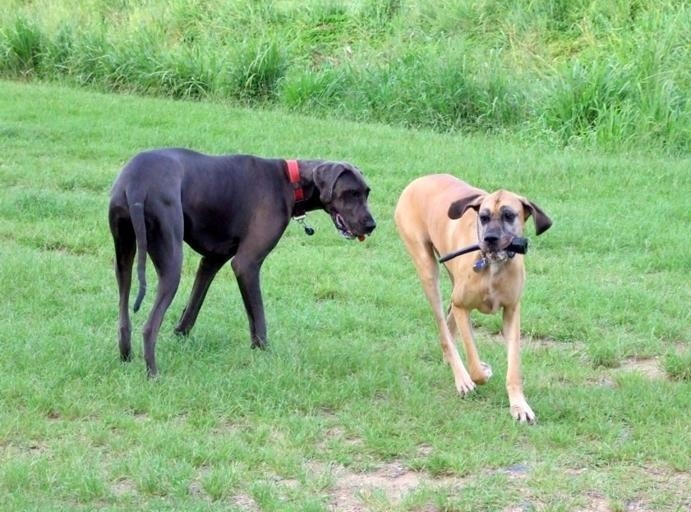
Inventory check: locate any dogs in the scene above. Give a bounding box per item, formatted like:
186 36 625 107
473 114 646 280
109 148 375 380
394 172 552 426
